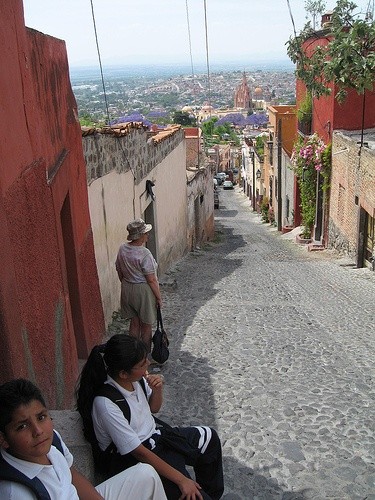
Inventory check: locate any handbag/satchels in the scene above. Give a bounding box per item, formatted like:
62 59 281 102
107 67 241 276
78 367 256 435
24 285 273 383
157 427 194 456
152 305 170 364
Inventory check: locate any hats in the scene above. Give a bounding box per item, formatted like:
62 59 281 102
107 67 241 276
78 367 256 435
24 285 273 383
127 218 152 241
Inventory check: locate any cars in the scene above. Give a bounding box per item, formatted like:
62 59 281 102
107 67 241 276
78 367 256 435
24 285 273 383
214 173 226 184
223 180 233 189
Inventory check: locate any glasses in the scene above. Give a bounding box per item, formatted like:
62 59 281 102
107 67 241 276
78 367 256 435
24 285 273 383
128 359 149 370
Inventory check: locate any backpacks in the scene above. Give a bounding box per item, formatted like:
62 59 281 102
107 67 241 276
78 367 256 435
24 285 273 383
77 378 147 457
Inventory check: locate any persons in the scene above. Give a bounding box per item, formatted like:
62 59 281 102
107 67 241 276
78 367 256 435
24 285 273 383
0 379 167 500
74 334 225 500
115 218 163 364
